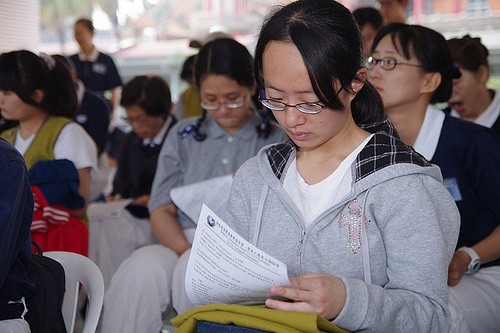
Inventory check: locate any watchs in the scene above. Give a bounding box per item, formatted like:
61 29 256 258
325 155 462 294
456 246 481 275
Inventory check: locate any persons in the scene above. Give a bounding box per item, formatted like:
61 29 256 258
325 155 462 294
216 0 462 333
178 54 208 117
53 54 111 200
98 37 288 333
0 49 98 257
377 0 408 27
86 74 178 292
68 17 123 132
365 21 500 333
440 33 500 131
352 6 383 58
0 137 34 333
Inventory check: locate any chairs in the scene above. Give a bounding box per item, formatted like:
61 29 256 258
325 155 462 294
41 250 105 333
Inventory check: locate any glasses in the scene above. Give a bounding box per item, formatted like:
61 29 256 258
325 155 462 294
199 86 250 110
258 86 345 114
366 56 423 72
120 110 149 124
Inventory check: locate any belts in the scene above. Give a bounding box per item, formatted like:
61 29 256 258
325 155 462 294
0 303 25 321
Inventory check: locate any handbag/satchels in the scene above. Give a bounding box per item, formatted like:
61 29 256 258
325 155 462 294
22 239 66 333
